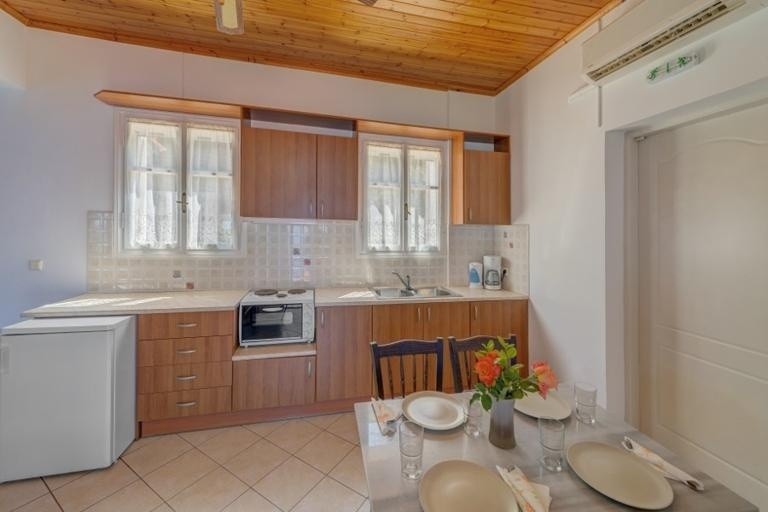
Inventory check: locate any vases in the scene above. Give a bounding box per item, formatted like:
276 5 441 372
488 388 519 454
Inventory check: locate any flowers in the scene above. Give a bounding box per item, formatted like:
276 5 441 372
463 335 564 410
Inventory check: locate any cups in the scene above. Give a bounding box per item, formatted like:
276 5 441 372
571 379 599 424
537 415 569 477
468 262 484 290
397 419 425 483
464 391 482 438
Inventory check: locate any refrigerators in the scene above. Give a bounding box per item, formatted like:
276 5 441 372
1 315 139 483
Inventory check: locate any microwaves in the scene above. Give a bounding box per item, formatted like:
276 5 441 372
239 288 315 348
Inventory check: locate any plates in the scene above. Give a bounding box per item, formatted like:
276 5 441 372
515 387 572 423
401 389 464 434
416 459 521 512
565 441 676 511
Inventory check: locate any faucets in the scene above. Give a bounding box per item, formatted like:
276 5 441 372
392 272 414 290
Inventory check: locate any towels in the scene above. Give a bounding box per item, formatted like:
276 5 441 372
367 395 407 438
491 460 558 511
617 432 715 497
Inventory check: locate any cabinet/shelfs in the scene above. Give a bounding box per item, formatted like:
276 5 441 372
467 295 531 381
230 351 319 430
234 103 360 224
369 297 475 401
313 300 374 417
132 305 239 441
449 125 513 229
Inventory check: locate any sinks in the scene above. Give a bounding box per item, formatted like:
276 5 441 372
369 285 414 304
415 285 463 304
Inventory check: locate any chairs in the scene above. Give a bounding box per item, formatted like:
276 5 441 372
366 334 447 402
445 327 519 397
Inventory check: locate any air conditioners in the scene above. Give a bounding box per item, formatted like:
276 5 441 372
578 1 745 84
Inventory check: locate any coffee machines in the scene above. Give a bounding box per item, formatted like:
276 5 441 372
483 255 502 290
